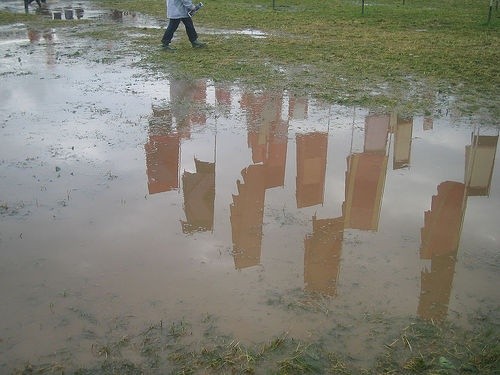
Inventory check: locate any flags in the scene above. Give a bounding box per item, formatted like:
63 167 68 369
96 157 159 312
145 80 498 328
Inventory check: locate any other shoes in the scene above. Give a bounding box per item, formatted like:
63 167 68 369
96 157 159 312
192 41 209 48
162 44 176 50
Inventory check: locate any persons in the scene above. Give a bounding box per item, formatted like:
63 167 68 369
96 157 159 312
36 0 46 8
159 0 207 48
